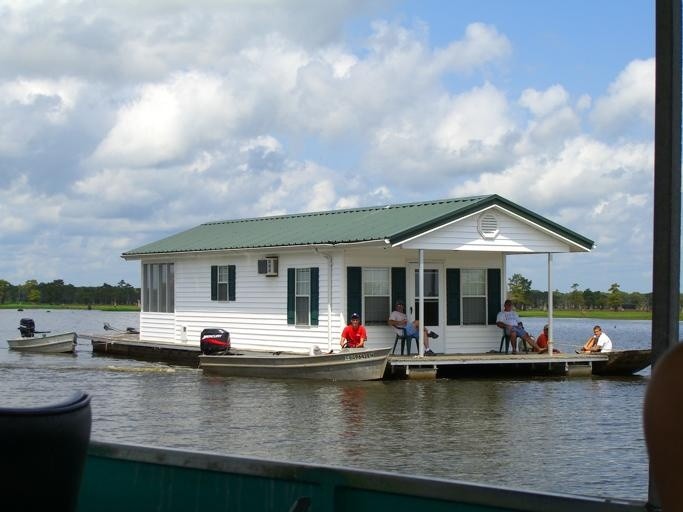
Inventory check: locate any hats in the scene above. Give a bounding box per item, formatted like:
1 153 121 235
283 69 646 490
352 313 360 319
505 300 512 304
544 324 550 329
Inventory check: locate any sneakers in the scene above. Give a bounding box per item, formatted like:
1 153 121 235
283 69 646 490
424 349 436 356
427 330 439 338
575 350 581 354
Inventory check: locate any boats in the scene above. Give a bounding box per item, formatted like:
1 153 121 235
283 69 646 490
581 347 653 380
7 319 76 355
194 327 393 381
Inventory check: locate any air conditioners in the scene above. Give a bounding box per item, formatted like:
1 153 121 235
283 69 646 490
255 256 281 278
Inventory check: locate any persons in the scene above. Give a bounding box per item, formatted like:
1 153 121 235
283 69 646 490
575 326 612 354
494 300 547 354
339 312 367 349
532 322 559 353
388 299 439 355
638 337 682 512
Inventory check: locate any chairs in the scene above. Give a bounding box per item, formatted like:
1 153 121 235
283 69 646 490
0 383 96 512
392 324 421 356
498 322 530 355
